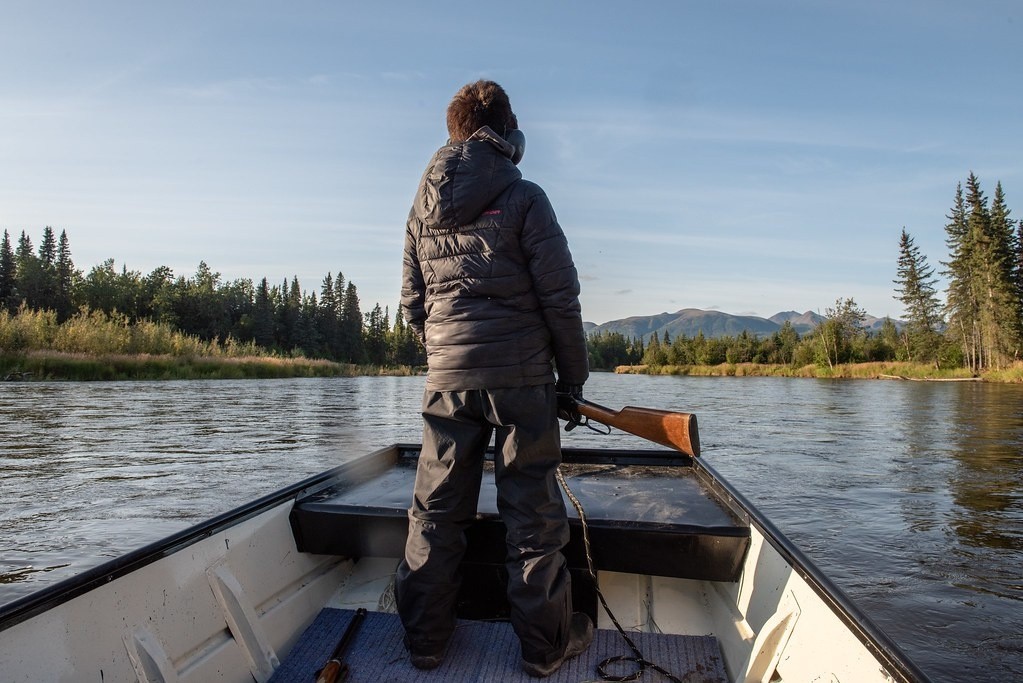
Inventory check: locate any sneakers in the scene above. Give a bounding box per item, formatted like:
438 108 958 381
411 618 458 671
521 611 595 679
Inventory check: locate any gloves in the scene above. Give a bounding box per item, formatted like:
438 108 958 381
555 379 583 432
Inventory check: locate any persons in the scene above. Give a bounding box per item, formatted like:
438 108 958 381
393 80 590 677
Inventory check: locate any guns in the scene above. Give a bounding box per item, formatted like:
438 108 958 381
555 391 701 460
